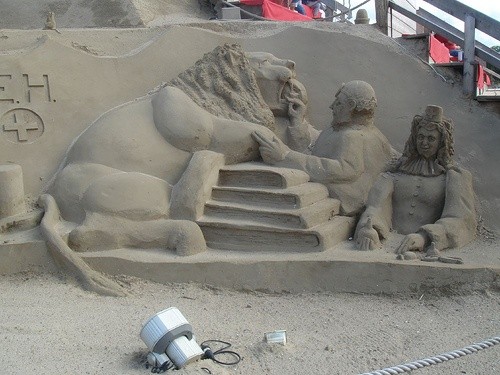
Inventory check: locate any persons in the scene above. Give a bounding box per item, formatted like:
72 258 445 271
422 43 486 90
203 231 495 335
284 0 305 15
353 104 479 252
301 0 326 19
433 33 464 59
249 79 378 182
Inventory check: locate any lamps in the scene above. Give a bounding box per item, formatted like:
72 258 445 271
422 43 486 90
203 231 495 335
136 306 205 371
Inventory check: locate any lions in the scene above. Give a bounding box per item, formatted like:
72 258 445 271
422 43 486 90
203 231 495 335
39 43 308 298
